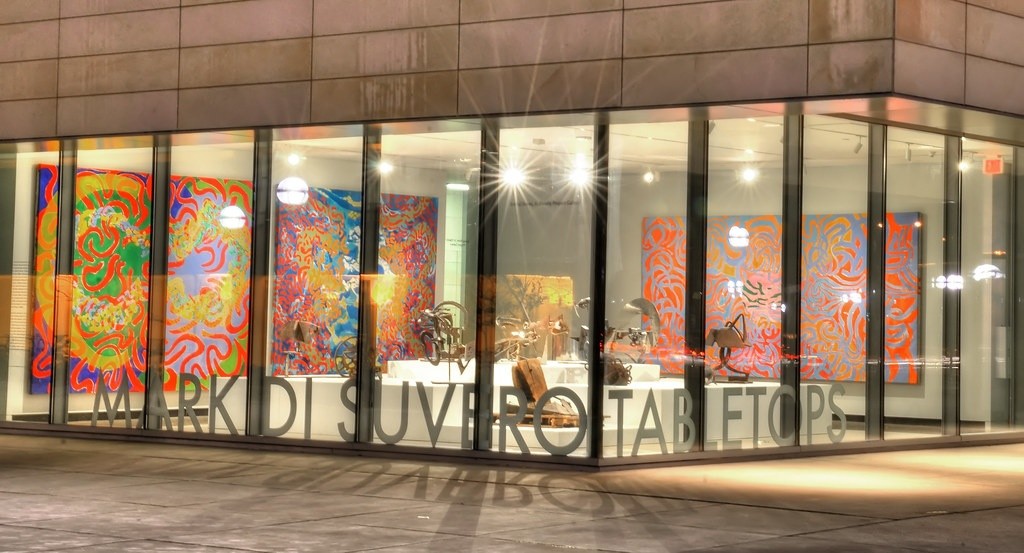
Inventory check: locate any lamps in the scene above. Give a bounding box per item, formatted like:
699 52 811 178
849 132 986 176
709 119 784 150
